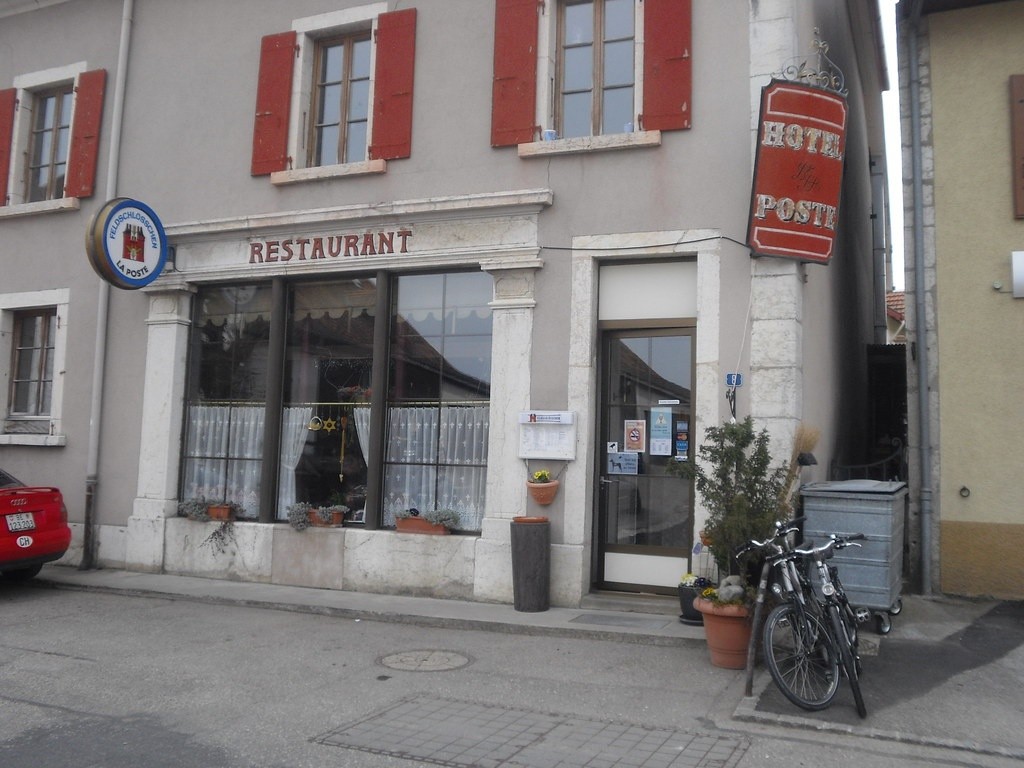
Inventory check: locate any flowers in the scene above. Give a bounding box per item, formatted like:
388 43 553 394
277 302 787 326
679 573 711 588
533 470 551 483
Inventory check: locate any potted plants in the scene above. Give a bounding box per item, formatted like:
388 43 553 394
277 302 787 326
395 507 459 535
285 501 351 531
666 415 802 672
178 495 249 554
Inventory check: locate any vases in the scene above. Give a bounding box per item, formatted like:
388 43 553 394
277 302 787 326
526 480 559 505
677 585 708 625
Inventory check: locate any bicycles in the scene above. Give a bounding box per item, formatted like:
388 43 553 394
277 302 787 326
734 515 872 718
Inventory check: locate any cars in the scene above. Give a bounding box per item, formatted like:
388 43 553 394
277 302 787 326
0 467 72 582
